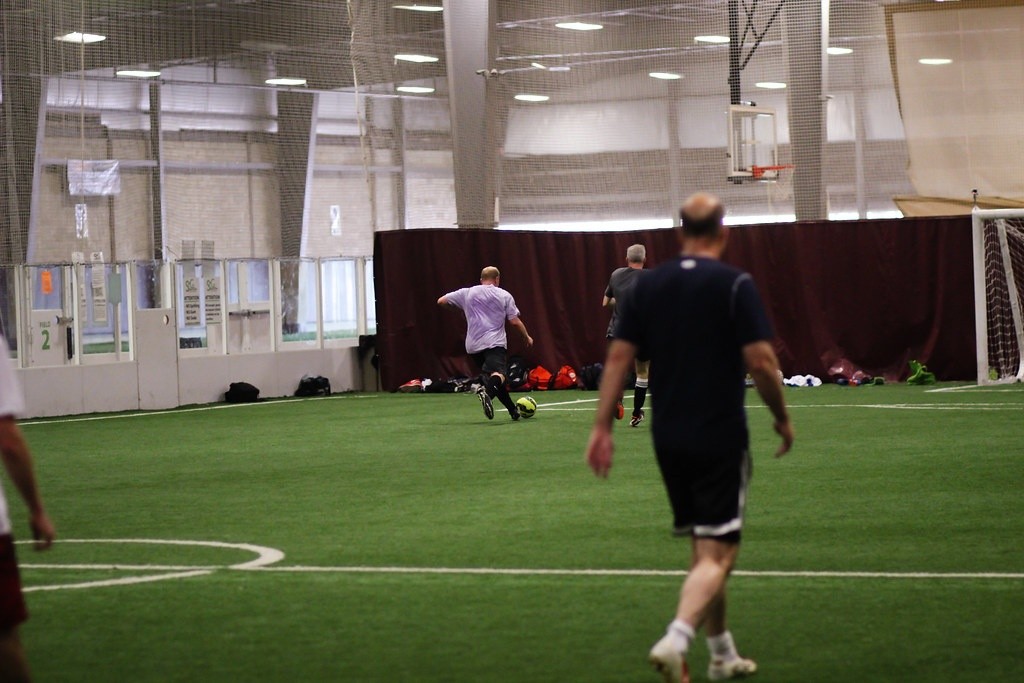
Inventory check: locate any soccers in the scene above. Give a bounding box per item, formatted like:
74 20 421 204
517 397 537 418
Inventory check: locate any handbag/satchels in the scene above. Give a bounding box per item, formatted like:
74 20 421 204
294 375 332 397
225 382 260 403
906 358 935 385
398 362 638 393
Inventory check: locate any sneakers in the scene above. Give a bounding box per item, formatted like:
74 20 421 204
650 639 690 683
708 656 758 682
630 410 644 426
509 405 520 420
614 392 623 420
478 392 494 420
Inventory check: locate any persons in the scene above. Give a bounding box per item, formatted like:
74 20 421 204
603 244 654 427
0 332 57 683
587 189 795 683
435 266 535 422
